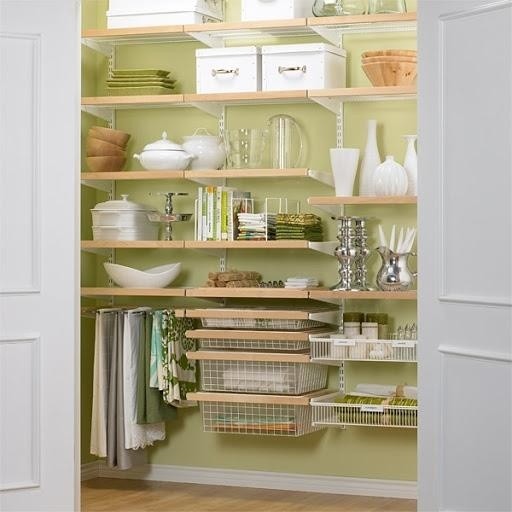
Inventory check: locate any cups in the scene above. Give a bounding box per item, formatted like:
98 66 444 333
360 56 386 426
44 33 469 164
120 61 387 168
329 148 360 196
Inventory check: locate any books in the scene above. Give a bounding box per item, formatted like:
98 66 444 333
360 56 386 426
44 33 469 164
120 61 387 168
197 186 252 241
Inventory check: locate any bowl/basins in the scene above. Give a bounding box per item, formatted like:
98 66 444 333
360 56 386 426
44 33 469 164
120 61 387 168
86 125 131 170
360 49 416 87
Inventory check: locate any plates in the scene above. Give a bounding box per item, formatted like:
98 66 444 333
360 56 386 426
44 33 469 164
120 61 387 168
105 70 177 95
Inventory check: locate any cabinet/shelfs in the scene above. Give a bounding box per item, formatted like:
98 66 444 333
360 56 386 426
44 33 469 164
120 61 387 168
80 1 420 497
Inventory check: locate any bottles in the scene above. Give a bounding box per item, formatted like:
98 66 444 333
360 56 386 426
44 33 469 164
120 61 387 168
360 119 381 196
374 156 408 197
401 135 417 197
328 311 389 359
